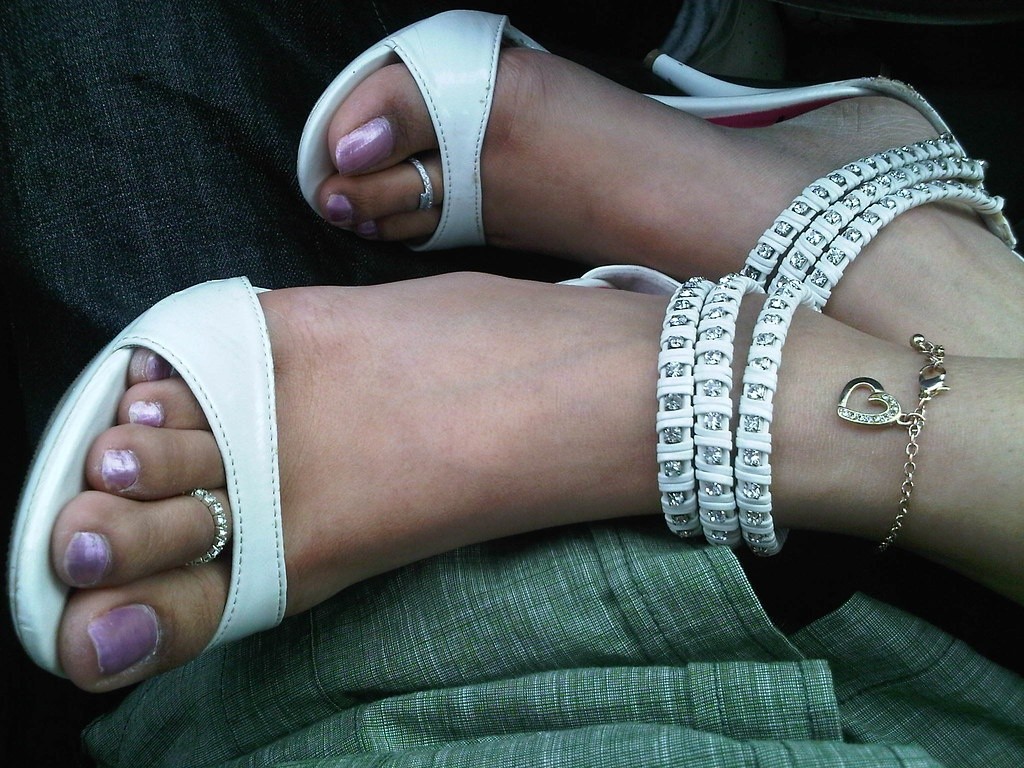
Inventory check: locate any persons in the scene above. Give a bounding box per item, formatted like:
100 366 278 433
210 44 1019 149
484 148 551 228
0 1 1024 768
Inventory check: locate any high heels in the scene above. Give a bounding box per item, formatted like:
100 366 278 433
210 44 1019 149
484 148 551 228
10 263 790 688
289 10 1024 310
